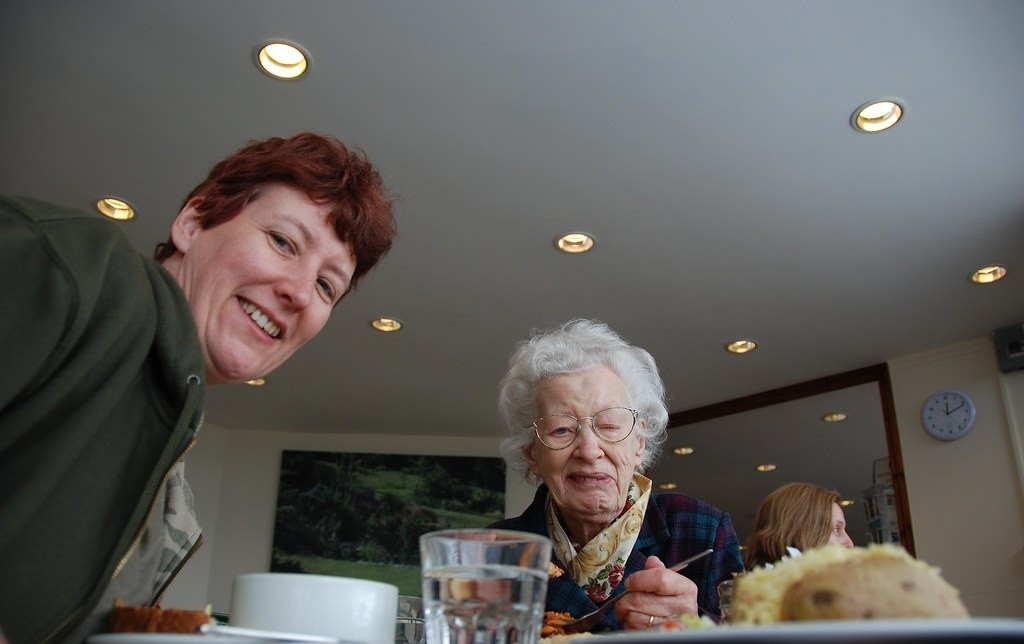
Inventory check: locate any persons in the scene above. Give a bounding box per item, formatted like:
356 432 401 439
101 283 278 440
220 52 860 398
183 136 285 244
0 132 397 644
433 320 746 632
747 482 856 568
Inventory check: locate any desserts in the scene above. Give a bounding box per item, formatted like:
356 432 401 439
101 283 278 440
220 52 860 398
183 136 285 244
110 599 210 633
727 539 971 626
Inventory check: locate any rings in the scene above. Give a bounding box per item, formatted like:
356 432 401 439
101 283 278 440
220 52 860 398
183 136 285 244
648 616 653 627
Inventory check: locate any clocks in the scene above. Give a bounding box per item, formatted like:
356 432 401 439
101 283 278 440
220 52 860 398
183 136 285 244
921 388 976 442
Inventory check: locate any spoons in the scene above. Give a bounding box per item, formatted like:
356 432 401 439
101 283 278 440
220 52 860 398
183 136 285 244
564 548 714 634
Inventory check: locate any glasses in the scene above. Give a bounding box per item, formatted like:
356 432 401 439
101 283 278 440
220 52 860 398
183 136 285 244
531 407 637 450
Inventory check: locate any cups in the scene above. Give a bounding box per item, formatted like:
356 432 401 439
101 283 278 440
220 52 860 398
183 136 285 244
418 527 554 643
393 595 427 644
227 573 399 644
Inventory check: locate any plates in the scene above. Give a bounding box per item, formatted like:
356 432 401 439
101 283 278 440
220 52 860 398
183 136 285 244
558 615 1023 644
87 633 338 644
